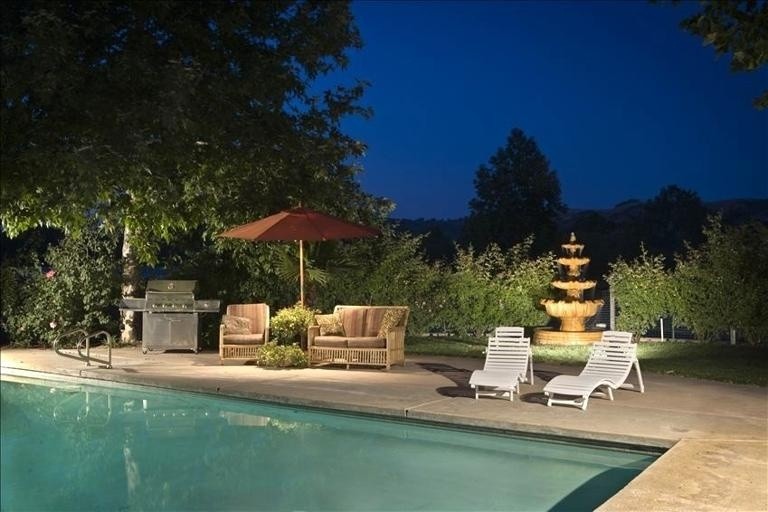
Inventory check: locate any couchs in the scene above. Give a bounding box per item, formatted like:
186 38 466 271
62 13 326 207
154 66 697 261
219 303 269 365
308 305 410 370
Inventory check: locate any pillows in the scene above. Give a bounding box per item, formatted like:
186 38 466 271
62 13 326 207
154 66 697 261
222 315 252 335
378 309 407 338
315 312 346 337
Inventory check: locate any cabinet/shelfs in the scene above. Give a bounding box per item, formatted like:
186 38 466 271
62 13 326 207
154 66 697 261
143 313 198 354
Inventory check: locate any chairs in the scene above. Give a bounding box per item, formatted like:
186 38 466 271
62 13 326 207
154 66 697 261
469 327 534 401
543 330 644 411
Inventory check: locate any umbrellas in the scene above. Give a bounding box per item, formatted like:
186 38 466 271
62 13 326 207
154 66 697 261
216 202 381 352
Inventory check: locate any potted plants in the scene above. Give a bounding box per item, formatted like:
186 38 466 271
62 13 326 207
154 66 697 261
256 338 308 369
